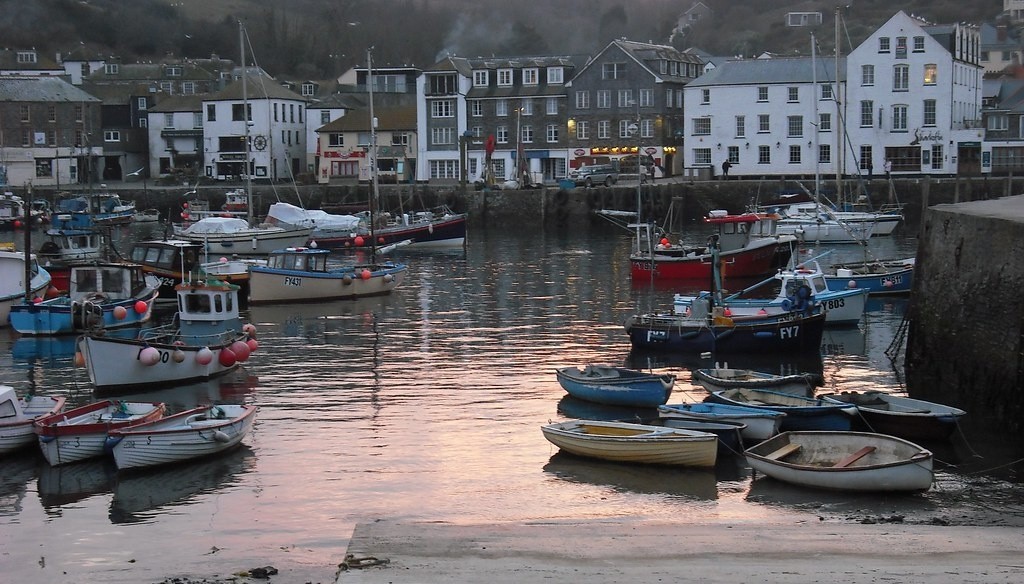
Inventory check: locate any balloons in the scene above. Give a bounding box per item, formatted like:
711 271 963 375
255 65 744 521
657 238 671 250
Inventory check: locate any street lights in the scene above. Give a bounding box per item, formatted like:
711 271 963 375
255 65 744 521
514 106 524 179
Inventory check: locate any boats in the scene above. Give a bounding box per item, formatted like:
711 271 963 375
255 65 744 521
673 260 871 326
0 385 68 453
34 400 170 467
817 389 970 440
712 388 858 434
555 364 677 411
625 233 830 358
611 416 748 450
656 402 787 441
692 368 819 400
744 430 935 494
540 417 719 470
107 405 258 472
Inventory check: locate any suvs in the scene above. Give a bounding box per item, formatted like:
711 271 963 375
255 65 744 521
568 164 619 187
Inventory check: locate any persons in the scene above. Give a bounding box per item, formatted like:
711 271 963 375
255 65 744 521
640 163 655 184
722 159 732 180
884 158 892 179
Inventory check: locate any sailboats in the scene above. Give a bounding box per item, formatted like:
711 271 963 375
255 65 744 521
596 8 916 292
0 21 470 399
